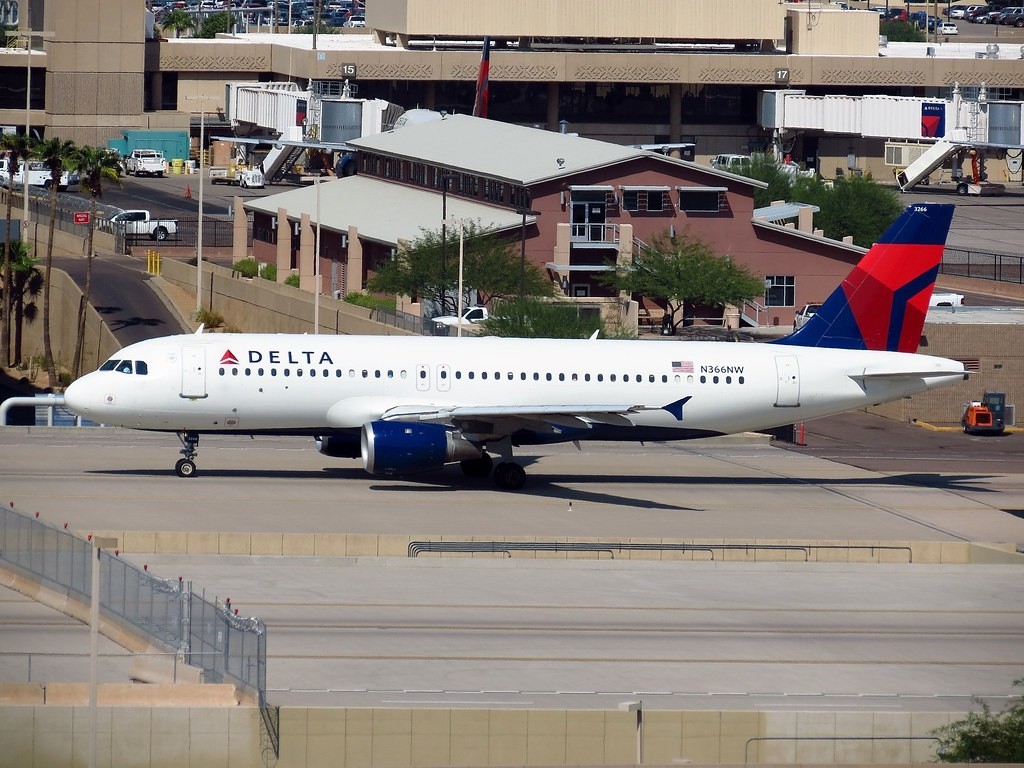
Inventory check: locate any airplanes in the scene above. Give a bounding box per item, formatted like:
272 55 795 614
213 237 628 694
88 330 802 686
67 199 981 477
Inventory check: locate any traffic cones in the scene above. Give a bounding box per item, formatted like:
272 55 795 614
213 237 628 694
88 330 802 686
185 185 193 199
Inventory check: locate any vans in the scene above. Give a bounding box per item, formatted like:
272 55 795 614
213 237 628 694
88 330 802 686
709 152 751 174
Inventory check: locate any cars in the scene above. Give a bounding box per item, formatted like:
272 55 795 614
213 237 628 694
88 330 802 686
153 0 366 27
832 0 1024 36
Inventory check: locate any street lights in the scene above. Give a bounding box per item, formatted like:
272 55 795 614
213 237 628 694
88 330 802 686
183 94 222 314
441 173 461 315
3 28 59 266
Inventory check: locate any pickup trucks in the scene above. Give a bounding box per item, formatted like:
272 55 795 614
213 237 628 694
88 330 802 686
0 147 167 192
94 209 179 240
432 305 509 328
